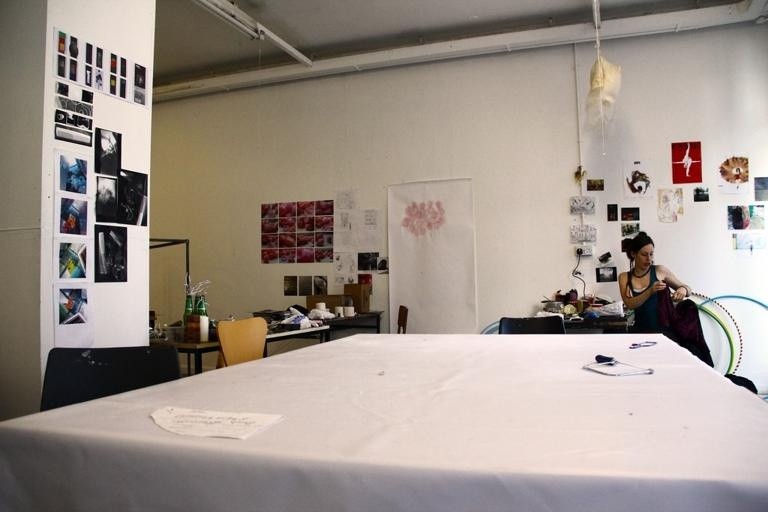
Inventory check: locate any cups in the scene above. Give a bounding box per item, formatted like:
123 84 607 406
315 303 325 311
336 306 354 317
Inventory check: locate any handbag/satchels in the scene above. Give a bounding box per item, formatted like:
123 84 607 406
622 272 636 333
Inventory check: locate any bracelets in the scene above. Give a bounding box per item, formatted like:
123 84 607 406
678 285 688 294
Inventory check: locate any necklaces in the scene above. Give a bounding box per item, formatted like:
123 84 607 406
630 266 651 278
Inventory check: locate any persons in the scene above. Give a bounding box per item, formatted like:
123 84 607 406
618 236 691 341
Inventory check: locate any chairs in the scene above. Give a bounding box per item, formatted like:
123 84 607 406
38 345 183 412
669 297 710 364
498 315 566 335
218 316 268 366
396 305 408 335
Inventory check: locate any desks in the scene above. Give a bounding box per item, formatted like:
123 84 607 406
560 315 629 333
1 333 768 511
163 325 331 375
324 311 386 345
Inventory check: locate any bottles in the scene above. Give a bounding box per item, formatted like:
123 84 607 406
183 294 206 325
